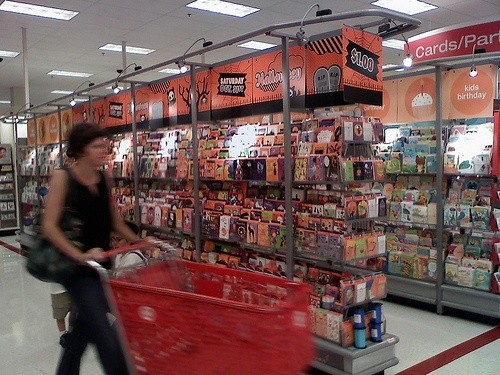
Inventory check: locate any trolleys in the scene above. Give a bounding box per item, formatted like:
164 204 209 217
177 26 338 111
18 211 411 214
85 239 313 375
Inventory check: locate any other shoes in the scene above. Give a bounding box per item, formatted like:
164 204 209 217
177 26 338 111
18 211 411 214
59 333 69 348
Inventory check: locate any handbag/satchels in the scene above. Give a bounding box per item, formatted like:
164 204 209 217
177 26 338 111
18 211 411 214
29 169 80 284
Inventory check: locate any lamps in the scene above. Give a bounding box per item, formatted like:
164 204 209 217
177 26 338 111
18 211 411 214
470 44 477 77
112 63 142 95
379 20 412 68
296 3 332 46
69 81 94 106
178 38 213 74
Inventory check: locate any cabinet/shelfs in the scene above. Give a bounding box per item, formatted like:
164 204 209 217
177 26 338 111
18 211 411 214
0 8 500 375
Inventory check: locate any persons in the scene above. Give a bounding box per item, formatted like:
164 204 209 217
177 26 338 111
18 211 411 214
0 146 6 158
42 122 145 375
112 220 147 279
50 282 77 348
